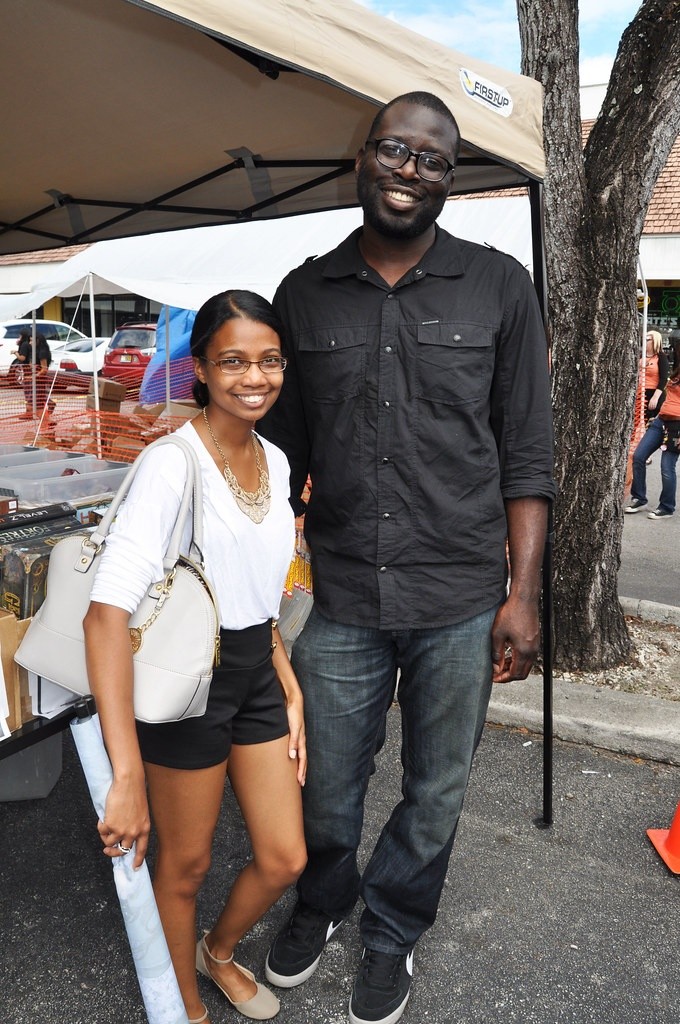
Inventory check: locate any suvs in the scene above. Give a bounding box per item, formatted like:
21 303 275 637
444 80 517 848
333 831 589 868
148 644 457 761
101 321 157 382
0 319 90 389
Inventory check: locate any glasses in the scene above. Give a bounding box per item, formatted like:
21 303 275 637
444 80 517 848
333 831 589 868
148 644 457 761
646 339 653 341
367 136 455 184
196 357 288 375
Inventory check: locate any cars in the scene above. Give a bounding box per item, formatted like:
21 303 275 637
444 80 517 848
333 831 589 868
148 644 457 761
49 337 112 389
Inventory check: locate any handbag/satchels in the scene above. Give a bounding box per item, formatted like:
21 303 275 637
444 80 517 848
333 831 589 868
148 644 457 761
663 418 680 453
13 435 221 723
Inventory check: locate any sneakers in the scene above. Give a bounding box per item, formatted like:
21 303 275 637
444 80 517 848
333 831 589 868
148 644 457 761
265 905 344 988
624 497 647 513
648 506 673 518
347 939 413 1024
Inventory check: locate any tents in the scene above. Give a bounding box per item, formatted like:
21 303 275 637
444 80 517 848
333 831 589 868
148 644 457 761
4 195 534 458
0 0 554 828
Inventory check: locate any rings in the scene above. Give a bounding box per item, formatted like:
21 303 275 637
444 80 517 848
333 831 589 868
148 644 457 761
118 842 133 853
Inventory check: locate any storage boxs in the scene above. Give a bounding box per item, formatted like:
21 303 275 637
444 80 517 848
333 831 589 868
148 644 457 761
0 444 134 504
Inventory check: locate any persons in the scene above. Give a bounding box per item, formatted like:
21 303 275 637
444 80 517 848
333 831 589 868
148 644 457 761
624 330 680 520
624 331 670 487
10 327 52 420
83 290 308 1024
254 91 558 1024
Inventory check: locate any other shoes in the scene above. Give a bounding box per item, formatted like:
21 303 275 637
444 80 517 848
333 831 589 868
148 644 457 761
194 935 280 1019
645 459 652 465
18 414 33 420
48 401 57 415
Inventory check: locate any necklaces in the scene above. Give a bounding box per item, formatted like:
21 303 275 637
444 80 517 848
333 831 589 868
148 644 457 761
202 403 271 524
646 354 654 363
